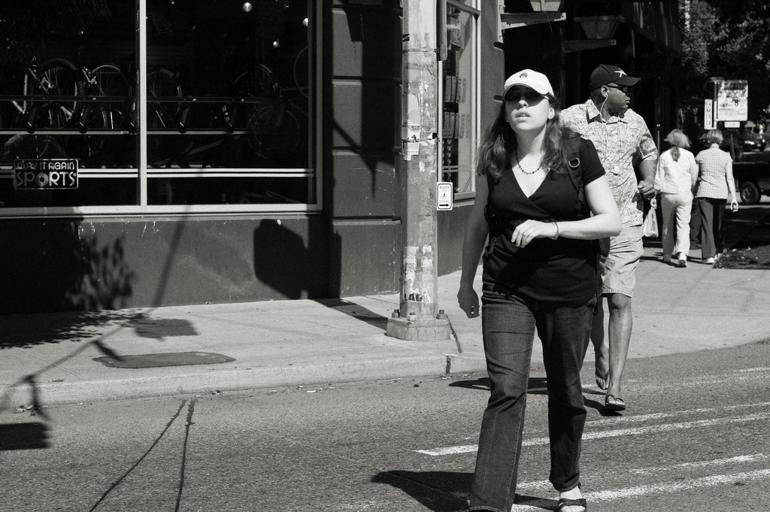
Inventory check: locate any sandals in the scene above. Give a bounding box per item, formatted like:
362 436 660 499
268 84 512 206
558 481 587 511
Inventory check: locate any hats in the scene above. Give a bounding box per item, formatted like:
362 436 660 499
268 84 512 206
503 68 554 98
588 64 642 90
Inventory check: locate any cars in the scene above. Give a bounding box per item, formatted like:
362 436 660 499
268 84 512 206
732 150 770 205
742 128 769 152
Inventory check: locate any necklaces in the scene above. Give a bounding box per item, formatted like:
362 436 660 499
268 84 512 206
514 146 545 175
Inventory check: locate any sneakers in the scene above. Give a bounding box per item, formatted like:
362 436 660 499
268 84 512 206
663 252 715 267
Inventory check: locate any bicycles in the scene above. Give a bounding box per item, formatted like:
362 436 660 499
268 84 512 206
0 30 308 168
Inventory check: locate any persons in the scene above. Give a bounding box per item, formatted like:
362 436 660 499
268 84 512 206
691 127 739 265
648 128 697 269
455 66 622 512
558 59 659 411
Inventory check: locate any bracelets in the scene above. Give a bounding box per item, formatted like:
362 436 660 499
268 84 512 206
553 220 561 243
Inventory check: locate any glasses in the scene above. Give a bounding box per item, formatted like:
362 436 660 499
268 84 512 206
606 84 631 94
504 91 551 103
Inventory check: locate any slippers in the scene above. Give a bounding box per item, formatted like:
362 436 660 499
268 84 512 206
593 368 611 390
605 393 626 412
